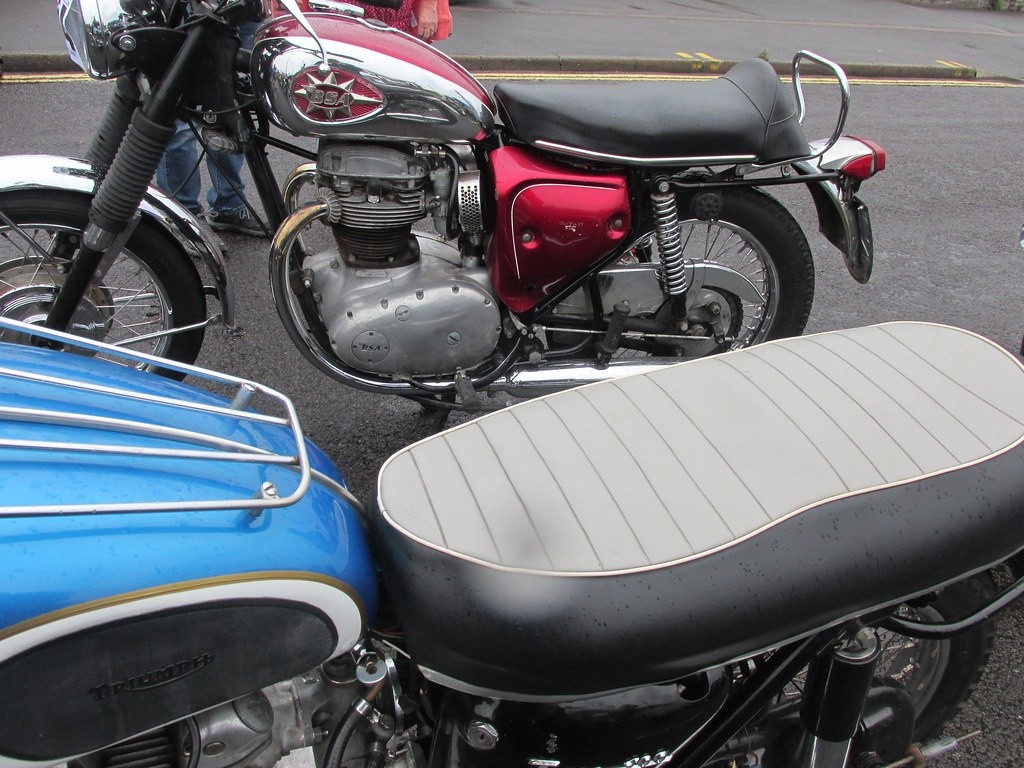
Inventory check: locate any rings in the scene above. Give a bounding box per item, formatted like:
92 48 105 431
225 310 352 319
430 32 434 34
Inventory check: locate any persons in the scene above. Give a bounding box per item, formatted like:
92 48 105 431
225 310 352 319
156 22 273 238
324 0 438 42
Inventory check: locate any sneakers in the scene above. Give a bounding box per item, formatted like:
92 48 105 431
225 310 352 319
165 212 228 256
205 202 272 237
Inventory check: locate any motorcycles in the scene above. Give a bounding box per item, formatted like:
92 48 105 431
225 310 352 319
0 1 887 442
0 315 1022 768
